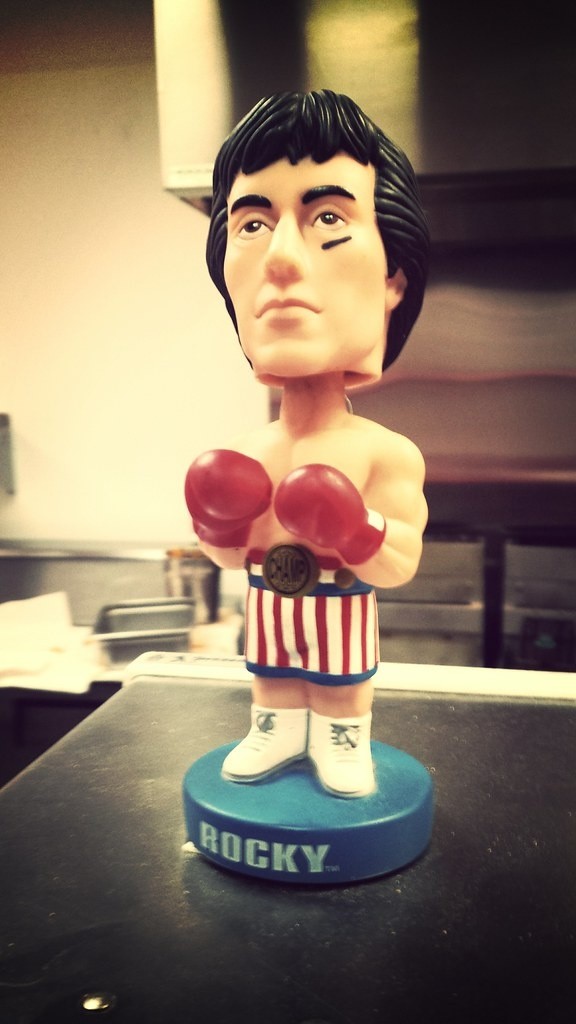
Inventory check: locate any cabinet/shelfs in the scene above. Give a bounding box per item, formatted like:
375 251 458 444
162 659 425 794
147 0 576 264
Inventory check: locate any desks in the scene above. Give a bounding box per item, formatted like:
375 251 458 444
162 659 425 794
1 675 576 1024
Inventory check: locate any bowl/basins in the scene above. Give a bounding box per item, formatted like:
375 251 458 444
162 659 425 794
89 597 195 662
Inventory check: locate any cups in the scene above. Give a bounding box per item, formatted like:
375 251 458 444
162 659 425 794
165 548 217 626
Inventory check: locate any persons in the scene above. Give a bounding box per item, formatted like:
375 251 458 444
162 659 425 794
184 88 431 804
